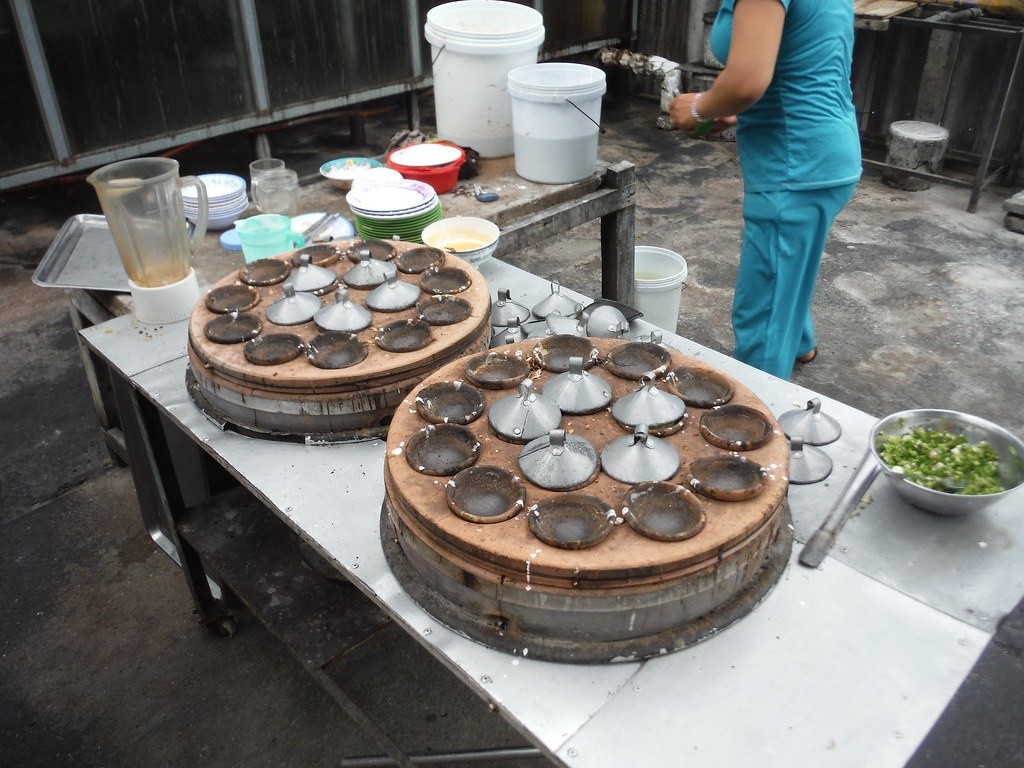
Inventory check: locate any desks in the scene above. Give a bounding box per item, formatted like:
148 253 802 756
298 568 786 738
63 154 636 468
76 256 1024 768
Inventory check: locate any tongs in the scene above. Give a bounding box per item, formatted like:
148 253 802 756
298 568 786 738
293 213 339 247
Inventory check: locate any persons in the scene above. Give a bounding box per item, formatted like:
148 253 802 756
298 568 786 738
667 0 863 381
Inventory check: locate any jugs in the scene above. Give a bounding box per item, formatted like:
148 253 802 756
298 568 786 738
234 214 305 265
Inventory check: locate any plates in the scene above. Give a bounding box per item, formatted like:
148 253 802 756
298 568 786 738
390 144 461 167
291 167 444 248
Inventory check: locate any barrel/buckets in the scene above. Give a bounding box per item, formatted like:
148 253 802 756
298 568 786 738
883 121 949 192
634 246 688 334
422 0 545 159
508 63 607 184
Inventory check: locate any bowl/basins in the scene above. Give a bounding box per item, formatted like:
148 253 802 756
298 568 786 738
422 217 500 264
870 409 1024 514
319 157 383 189
386 143 466 194
183 174 248 230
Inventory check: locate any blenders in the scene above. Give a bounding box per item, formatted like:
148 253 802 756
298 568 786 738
86 157 209 325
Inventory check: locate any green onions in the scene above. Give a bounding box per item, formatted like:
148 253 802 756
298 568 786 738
875 417 1019 495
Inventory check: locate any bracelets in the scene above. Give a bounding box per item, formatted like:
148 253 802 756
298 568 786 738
691 93 704 122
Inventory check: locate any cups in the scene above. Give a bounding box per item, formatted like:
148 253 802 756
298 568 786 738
257 169 298 224
250 158 285 212
